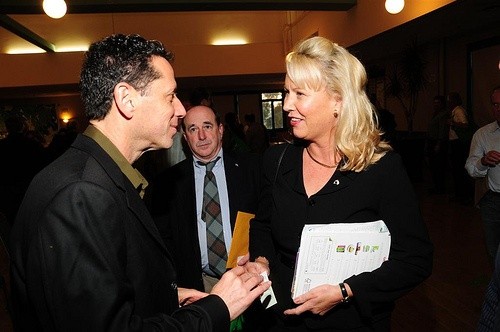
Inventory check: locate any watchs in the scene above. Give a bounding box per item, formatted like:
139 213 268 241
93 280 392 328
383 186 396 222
339 283 352 304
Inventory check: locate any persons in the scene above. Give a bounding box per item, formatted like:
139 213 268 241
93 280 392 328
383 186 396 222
9 32 274 332
475 243 500 332
237 36 435 332
464 87 500 268
0 105 160 225
149 105 279 332
369 93 473 209
164 86 270 168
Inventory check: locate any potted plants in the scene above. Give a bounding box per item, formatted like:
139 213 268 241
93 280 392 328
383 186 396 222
389 34 429 187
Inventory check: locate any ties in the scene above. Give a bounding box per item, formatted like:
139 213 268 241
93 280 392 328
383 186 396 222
196 156 229 280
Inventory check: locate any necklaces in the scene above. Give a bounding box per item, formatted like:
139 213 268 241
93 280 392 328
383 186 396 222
305 147 338 168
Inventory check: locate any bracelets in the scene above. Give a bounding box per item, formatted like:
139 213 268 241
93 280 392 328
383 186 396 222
481 157 488 166
